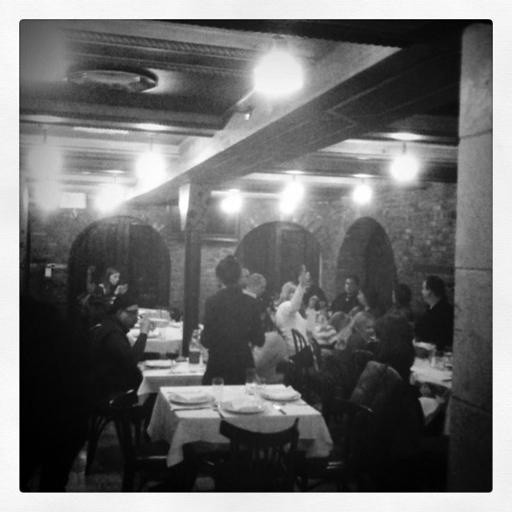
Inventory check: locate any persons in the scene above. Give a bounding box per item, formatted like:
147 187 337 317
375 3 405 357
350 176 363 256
333 275 361 312
83 267 128 311
355 285 385 317
201 255 266 386
68 291 156 441
244 273 267 299
274 265 316 370
354 313 426 491
296 266 315 289
237 268 251 286
334 312 376 470
302 287 329 321
417 277 450 344
380 284 414 329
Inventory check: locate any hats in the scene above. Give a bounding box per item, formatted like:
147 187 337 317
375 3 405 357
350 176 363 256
107 294 137 314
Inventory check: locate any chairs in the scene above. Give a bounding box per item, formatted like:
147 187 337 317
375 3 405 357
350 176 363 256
111 410 170 492
279 396 362 492
291 328 322 405
166 441 245 492
220 417 299 492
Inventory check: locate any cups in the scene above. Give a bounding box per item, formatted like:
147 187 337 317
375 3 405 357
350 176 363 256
167 349 180 373
245 376 257 395
212 377 224 407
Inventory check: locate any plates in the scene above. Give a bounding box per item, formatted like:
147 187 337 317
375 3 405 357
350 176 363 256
260 388 301 401
146 359 171 367
223 398 268 414
172 395 215 405
